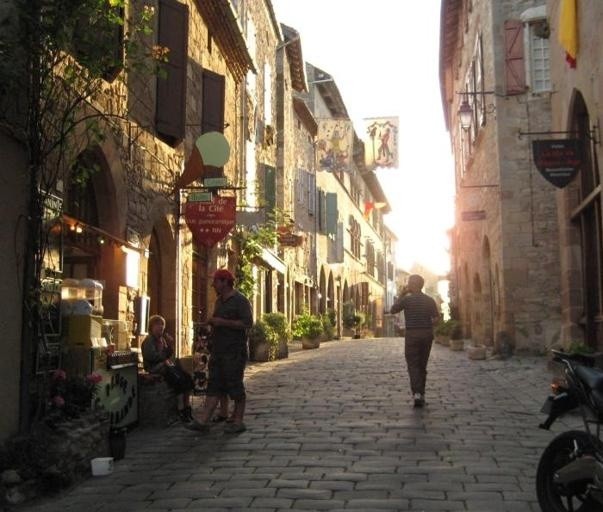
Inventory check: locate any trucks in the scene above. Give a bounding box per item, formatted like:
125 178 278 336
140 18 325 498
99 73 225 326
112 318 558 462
394 293 441 337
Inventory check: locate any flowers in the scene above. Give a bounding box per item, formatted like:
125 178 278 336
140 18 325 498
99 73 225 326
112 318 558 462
44 368 103 429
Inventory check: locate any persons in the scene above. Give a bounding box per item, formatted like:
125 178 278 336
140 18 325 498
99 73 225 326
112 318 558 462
141 315 195 422
185 270 253 434
209 394 231 422
376 128 390 161
391 273 440 406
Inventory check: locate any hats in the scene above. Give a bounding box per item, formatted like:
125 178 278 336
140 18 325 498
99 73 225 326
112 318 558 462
208 270 232 279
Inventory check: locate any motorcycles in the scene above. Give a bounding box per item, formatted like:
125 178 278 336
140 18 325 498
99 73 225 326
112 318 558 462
535 348 602 512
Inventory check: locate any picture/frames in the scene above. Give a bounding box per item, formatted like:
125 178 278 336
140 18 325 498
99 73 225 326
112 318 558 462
140 295 151 337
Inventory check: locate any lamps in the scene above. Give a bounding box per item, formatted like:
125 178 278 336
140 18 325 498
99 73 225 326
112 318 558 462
455 90 493 132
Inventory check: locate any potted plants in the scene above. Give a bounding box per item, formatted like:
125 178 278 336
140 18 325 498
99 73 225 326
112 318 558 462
433 319 487 360
248 306 373 362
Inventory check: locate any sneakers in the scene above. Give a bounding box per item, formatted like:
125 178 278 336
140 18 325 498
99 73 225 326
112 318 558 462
184 420 209 431
224 424 245 433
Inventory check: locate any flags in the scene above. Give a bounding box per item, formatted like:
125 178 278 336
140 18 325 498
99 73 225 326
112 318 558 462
558 0 579 69
315 120 354 173
364 117 399 172
